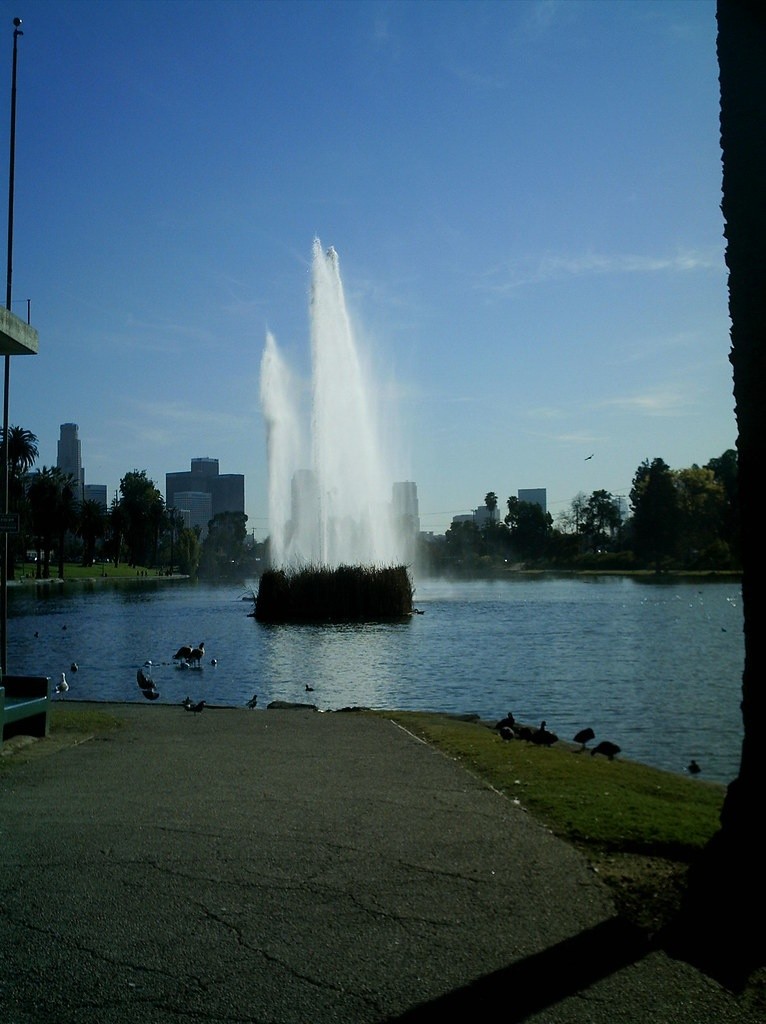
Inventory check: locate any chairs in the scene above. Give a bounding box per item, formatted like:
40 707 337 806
0 667 51 752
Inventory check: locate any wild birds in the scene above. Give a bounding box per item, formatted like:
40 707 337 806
136 660 159 700
305 685 314 691
54 673 70 694
70 661 80 673
62 625 68 630
171 643 219 671
585 452 594 460
182 696 207 715
34 631 39 638
492 713 622 759
245 695 257 710
687 760 701 778
